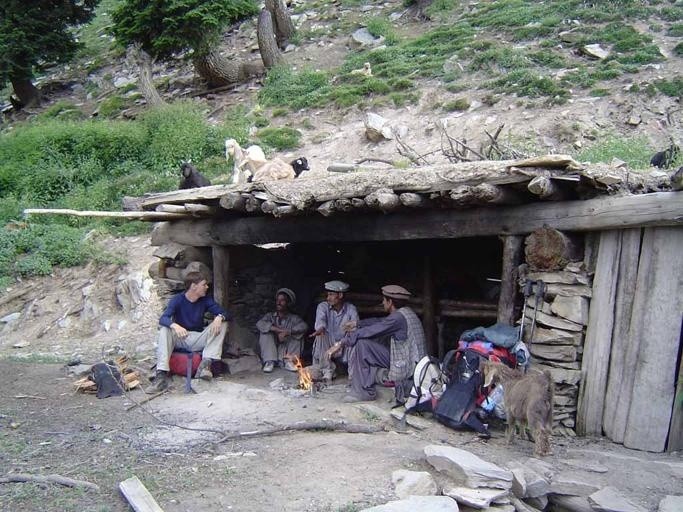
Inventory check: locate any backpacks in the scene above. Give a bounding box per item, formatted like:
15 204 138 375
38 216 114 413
405 354 442 412
437 349 491 430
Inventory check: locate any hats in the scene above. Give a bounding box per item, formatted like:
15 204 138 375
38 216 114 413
381 284 411 300
324 281 351 293
276 288 297 306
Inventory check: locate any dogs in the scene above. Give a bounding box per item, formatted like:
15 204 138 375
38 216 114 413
348 62 373 77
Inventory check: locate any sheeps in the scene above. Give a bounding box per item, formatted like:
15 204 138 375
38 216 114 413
177 162 211 190
224 139 265 184
238 157 311 183
482 358 554 457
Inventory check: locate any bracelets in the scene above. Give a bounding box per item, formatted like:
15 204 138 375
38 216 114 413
333 341 343 349
217 313 225 320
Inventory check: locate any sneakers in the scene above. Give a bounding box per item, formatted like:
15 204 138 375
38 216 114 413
262 361 275 373
344 384 374 391
284 359 298 372
341 392 375 402
320 370 336 381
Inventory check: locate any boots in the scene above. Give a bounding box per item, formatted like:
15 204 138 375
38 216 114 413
194 358 214 380
146 370 168 394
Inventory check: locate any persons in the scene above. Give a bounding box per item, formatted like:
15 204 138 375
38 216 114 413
307 279 360 379
143 270 231 395
255 286 309 372
323 284 427 404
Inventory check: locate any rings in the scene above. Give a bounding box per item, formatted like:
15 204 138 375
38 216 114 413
328 352 332 356
